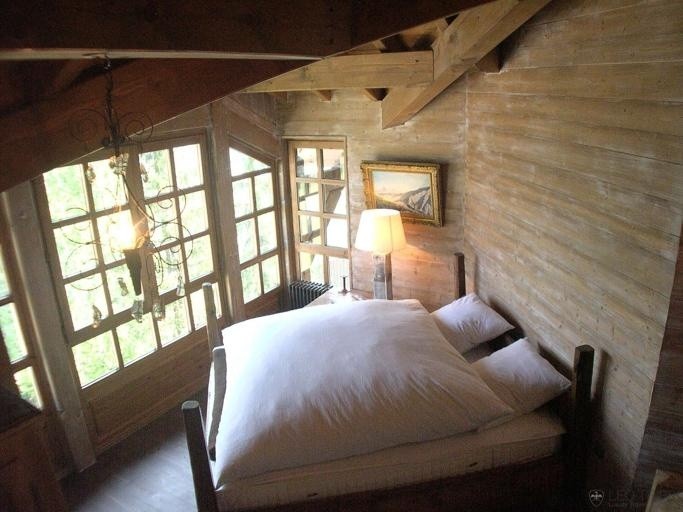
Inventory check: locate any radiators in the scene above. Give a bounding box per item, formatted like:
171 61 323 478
287 278 333 309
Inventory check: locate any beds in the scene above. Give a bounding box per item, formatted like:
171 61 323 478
181 251 598 511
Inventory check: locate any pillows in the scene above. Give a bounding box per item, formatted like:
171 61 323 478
468 336 572 432
212 293 516 494
429 289 515 355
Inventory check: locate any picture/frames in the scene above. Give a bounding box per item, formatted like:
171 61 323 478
360 158 444 229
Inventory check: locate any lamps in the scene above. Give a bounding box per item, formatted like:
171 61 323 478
354 208 407 299
53 56 196 332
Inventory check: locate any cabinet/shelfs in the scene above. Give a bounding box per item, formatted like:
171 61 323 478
0 386 71 512
